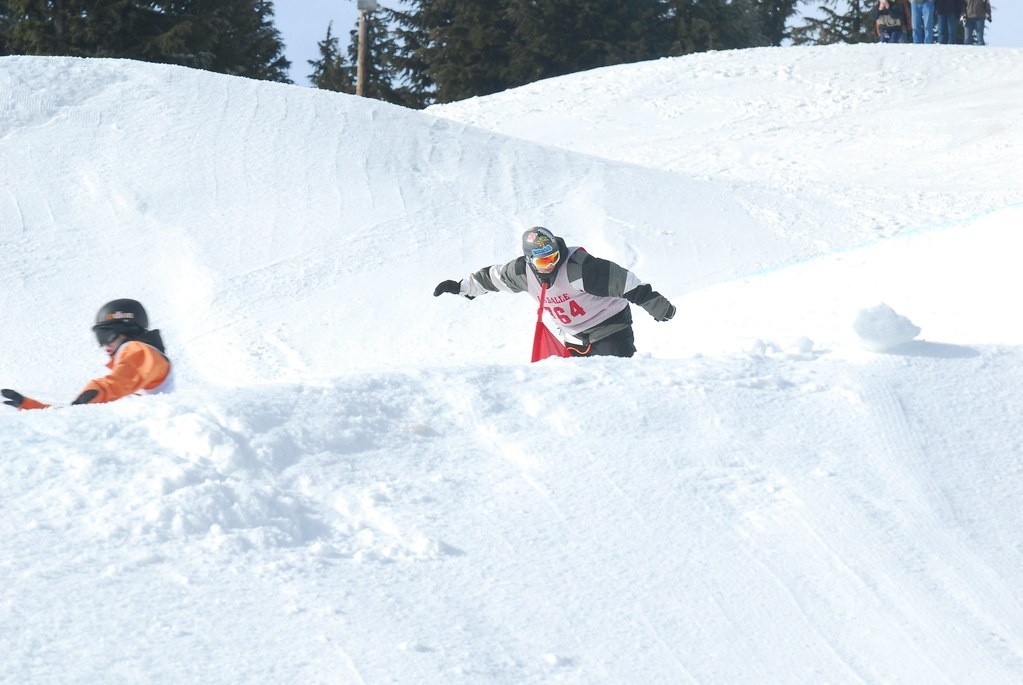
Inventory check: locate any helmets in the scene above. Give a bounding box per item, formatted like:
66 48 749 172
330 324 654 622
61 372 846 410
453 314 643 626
92 299 148 337
522 226 559 258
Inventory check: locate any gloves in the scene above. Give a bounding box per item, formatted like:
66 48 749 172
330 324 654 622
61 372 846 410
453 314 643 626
432 279 464 297
1 389 24 408
661 304 676 322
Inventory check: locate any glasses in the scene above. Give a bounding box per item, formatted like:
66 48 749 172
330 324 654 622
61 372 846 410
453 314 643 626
534 251 558 268
96 329 120 347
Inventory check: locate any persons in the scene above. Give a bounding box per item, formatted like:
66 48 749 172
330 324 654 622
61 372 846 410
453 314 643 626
1 298 176 412
433 226 677 357
